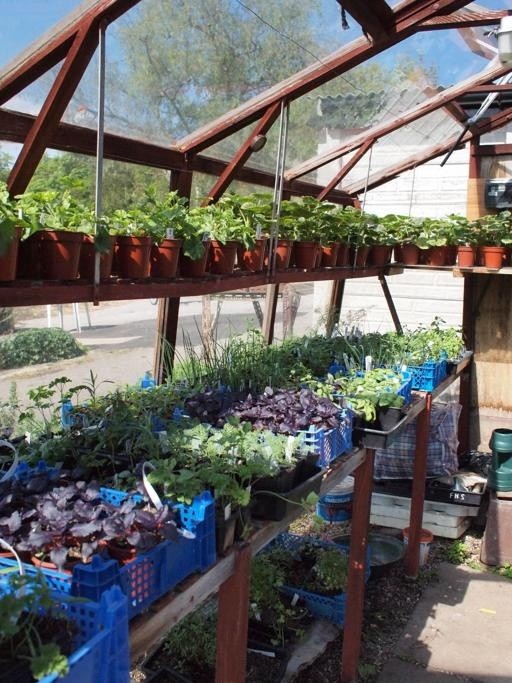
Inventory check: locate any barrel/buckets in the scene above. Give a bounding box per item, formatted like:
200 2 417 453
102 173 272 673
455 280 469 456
489 429 512 492
316 476 356 524
402 525 434 567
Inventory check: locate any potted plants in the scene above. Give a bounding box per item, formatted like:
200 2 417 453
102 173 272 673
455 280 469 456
0 318 469 682
0 182 512 280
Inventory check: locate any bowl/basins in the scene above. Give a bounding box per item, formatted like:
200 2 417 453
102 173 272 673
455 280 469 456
331 534 406 578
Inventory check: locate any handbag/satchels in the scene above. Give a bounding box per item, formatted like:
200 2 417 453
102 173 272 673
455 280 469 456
374 401 463 480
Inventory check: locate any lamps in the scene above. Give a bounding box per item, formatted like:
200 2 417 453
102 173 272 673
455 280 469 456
497 15 512 63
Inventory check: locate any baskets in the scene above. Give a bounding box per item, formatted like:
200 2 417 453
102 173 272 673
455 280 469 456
326 349 446 410
0 358 369 683
